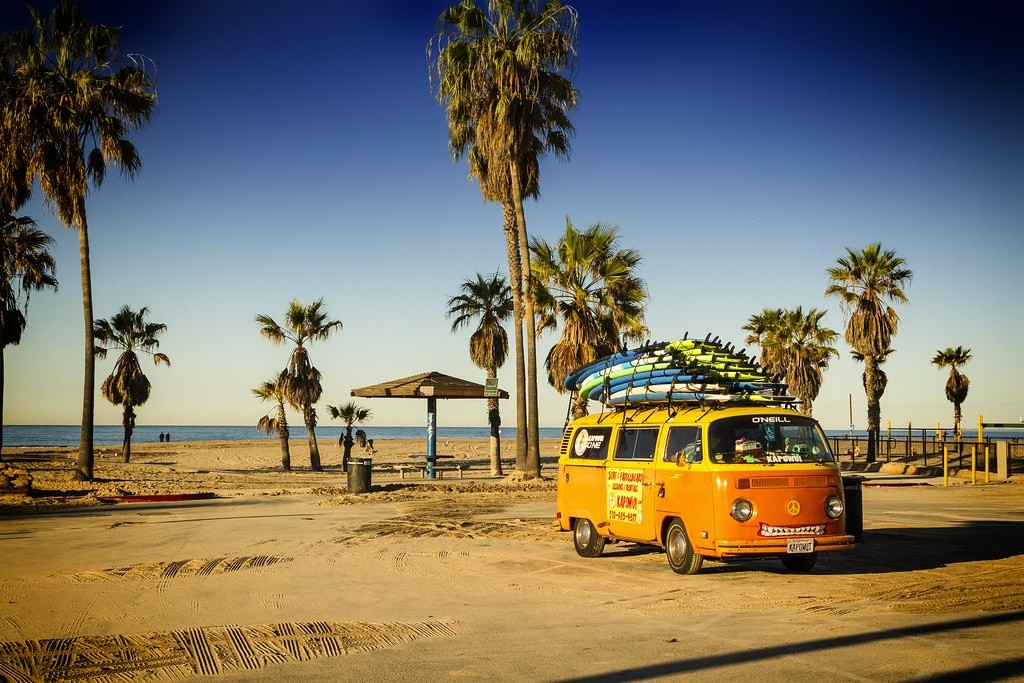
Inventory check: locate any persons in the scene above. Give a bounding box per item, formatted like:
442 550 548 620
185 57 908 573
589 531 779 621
159 432 171 443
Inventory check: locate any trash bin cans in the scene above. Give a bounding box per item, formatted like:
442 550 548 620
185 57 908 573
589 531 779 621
347 457 372 494
842 476 865 543
359 438 366 447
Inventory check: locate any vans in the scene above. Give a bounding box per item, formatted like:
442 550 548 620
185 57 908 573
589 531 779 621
556 405 856 575
